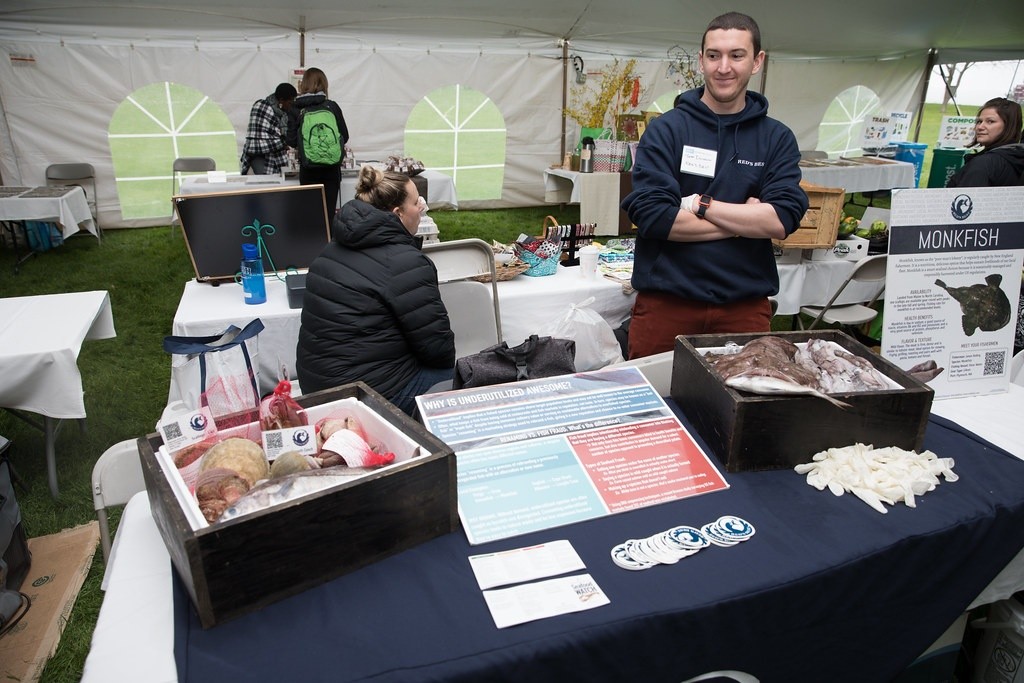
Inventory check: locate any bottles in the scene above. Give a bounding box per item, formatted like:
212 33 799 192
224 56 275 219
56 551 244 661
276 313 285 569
240 243 267 305
579 137 596 173
572 148 583 171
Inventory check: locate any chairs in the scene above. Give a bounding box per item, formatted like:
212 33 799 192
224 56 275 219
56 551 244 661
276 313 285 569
415 238 503 356
92 438 143 572
47 164 107 242
796 254 887 332
169 157 216 239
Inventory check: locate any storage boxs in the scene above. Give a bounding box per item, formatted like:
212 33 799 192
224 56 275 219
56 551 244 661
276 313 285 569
135 380 461 628
774 181 848 248
671 332 936 477
808 233 871 261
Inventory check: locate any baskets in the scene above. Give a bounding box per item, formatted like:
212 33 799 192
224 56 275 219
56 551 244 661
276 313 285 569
471 247 531 283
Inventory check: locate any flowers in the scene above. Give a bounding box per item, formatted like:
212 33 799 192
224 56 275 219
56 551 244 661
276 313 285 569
556 52 640 126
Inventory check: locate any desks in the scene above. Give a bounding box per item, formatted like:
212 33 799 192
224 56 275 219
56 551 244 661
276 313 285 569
0 290 117 507
0 183 100 276
167 226 887 424
172 356 1024 683
292 158 456 213
541 155 915 237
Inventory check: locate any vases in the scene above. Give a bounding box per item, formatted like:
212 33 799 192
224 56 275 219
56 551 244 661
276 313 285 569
578 129 610 154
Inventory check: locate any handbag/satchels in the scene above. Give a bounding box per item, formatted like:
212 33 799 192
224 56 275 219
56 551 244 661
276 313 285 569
164 318 265 418
593 124 627 173
453 336 576 392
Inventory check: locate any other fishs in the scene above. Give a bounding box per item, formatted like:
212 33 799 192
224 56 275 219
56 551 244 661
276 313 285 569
934 272 1011 338
213 447 421 525
700 334 881 407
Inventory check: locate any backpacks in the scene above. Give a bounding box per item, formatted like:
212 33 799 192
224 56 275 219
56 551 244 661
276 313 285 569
294 100 346 168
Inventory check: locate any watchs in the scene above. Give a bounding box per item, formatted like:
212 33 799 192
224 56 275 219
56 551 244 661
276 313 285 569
696 193 713 220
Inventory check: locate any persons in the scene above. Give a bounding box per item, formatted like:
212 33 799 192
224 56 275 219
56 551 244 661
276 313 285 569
619 11 810 361
241 67 349 242
295 165 457 419
946 98 1024 188
